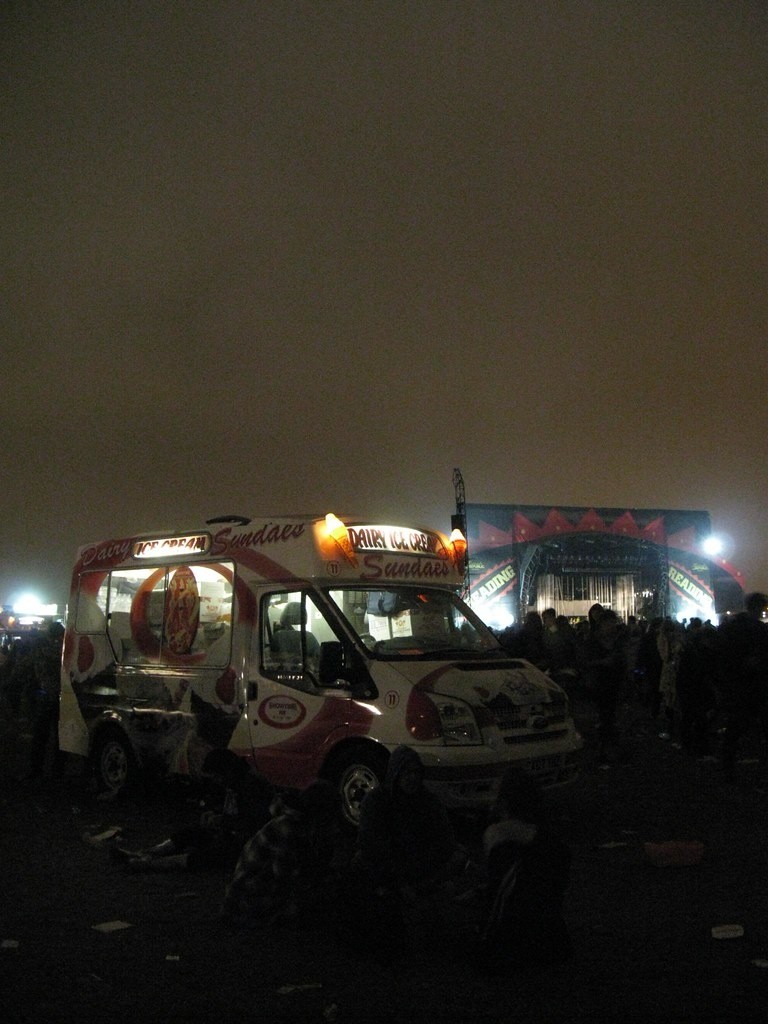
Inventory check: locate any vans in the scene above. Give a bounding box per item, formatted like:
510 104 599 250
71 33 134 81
54 517 576 842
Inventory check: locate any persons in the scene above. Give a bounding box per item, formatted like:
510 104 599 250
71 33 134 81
496 591 768 771
111 749 573 963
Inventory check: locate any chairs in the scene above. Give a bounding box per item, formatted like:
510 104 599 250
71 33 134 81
270 630 321 680
